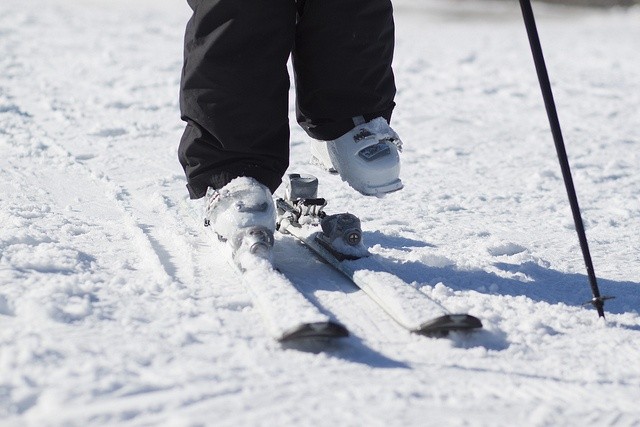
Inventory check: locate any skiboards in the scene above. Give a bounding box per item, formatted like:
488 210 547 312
212 192 484 341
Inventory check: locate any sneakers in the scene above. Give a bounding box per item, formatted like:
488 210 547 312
203 176 274 236
327 124 400 187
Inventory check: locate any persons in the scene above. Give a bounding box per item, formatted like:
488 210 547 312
175 0 405 241
